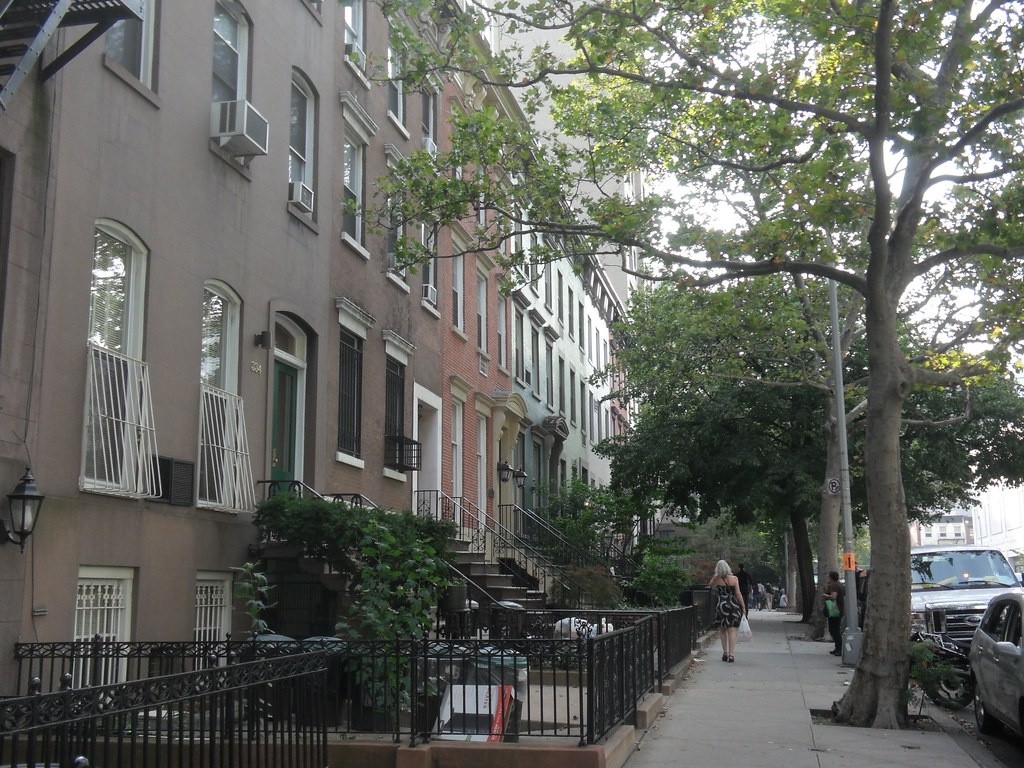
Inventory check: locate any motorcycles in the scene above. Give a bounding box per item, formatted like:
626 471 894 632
910 613 976 709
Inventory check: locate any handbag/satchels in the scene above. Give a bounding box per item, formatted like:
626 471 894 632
736 614 753 642
825 595 841 618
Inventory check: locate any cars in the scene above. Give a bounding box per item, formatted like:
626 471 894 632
969 594 1024 733
856 570 869 630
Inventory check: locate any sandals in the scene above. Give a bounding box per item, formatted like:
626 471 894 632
722 652 728 661
729 655 734 662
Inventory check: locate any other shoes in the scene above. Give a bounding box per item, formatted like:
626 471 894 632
830 648 841 656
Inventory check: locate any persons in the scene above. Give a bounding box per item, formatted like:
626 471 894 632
708 560 746 661
734 563 752 619
747 580 790 612
855 561 867 599
822 571 845 656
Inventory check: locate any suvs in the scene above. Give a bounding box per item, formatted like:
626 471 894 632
911 544 1023 641
